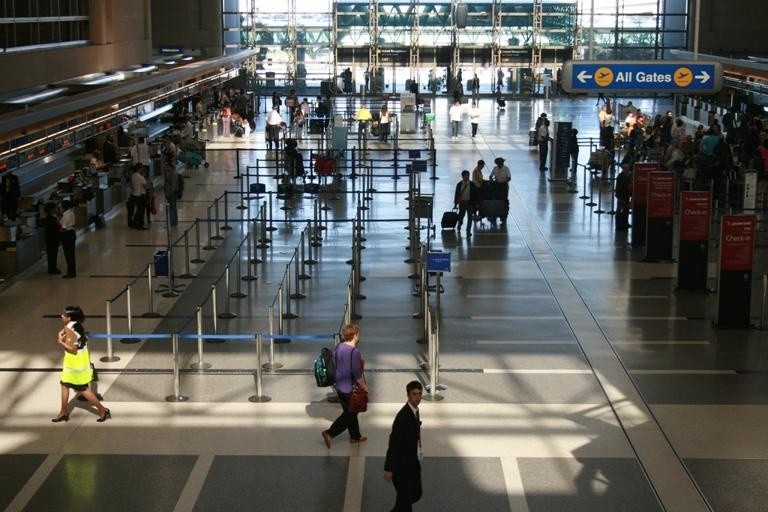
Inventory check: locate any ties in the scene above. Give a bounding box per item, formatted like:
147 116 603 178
414 411 421 448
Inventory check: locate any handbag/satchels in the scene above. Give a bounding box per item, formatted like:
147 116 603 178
348 388 368 414
455 182 469 204
150 192 157 214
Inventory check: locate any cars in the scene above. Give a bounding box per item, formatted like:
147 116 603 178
535 73 545 84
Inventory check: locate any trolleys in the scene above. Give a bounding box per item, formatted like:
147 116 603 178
477 180 508 220
587 146 613 170
184 138 212 169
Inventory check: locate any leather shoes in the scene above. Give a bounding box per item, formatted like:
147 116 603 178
322 431 332 448
350 436 367 443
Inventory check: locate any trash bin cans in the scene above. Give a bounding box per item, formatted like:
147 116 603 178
154 250 168 276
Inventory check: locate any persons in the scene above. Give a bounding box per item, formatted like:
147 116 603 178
536 113 579 172
322 324 367 447
2 172 20 221
266 65 393 175
455 157 512 236
411 68 562 108
44 64 256 278
385 381 422 510
597 86 768 215
51 305 111 421
449 100 479 139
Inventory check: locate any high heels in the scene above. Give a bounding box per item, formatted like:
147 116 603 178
96 408 111 422
52 415 68 422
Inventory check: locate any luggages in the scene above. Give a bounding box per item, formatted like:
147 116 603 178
480 200 509 217
480 181 508 201
440 207 458 229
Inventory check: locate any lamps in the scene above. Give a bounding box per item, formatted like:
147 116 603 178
140 103 173 123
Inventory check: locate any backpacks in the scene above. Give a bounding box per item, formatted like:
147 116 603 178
312 346 336 387
175 174 184 199
